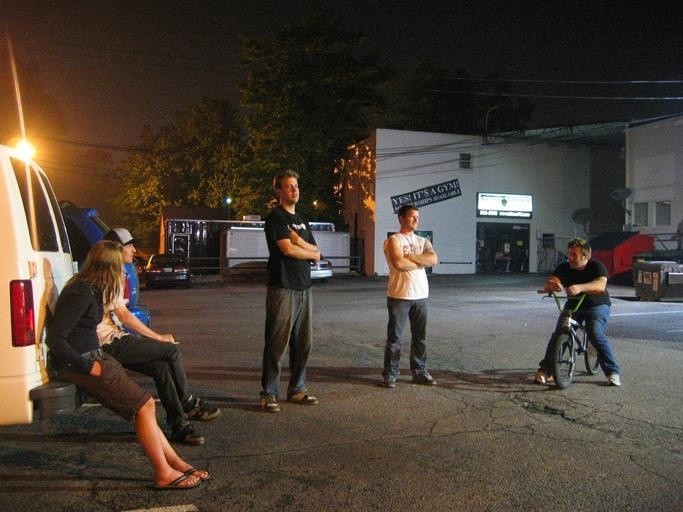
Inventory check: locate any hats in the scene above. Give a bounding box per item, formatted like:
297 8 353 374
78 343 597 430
102 227 140 246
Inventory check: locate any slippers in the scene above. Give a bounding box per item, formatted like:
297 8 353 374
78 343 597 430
151 466 210 489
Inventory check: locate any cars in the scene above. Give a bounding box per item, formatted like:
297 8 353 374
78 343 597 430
309 258 334 284
143 253 192 289
131 256 148 290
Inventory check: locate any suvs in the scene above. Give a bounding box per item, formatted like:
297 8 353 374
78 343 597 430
51 195 154 339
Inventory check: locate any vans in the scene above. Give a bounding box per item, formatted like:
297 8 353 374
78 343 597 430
0 146 84 430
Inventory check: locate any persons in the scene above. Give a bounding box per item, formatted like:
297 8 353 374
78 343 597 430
382 204 437 389
42 239 212 490
533 237 622 386
97 226 222 445
259 170 321 414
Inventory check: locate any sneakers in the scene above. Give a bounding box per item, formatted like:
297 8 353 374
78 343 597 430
606 374 620 386
184 395 222 420
382 376 396 387
260 391 280 412
286 393 319 405
171 423 205 445
413 373 436 385
533 371 553 383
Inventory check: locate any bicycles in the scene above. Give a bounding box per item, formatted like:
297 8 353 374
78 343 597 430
536 286 603 389
